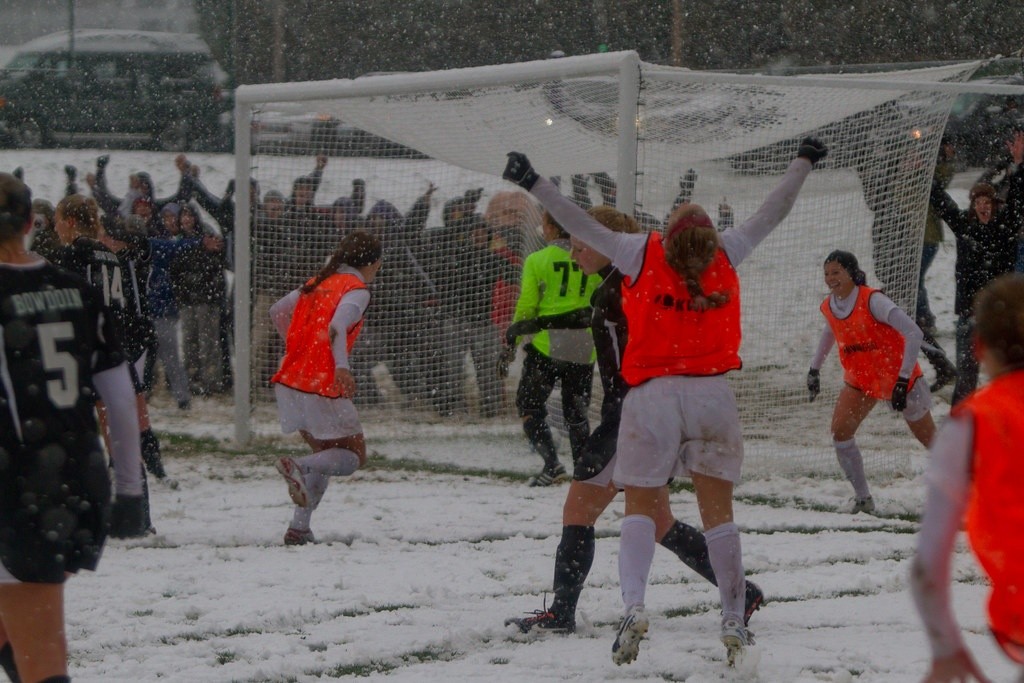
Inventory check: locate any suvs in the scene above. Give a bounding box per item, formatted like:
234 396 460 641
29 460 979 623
0 27 236 150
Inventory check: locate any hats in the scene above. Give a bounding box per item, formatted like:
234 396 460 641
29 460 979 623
968 183 1007 203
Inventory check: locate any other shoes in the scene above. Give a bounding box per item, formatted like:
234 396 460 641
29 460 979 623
929 359 960 392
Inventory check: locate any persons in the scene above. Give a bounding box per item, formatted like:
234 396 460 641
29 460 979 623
500 133 829 666
499 204 767 633
1 169 158 683
266 232 382 543
322 164 732 422
854 118 1023 406
906 270 1023 683
0 153 327 496
490 207 607 487
805 249 938 512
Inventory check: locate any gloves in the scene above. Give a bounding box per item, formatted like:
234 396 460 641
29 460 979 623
798 137 829 165
503 151 541 192
505 318 542 347
806 367 821 402
892 376 910 412
111 494 153 539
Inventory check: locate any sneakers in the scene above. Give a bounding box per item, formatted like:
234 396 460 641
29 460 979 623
285 530 316 547
837 496 875 515
720 610 756 668
529 462 571 486
593 605 648 667
744 580 766 627
276 457 311 509
503 590 576 636
